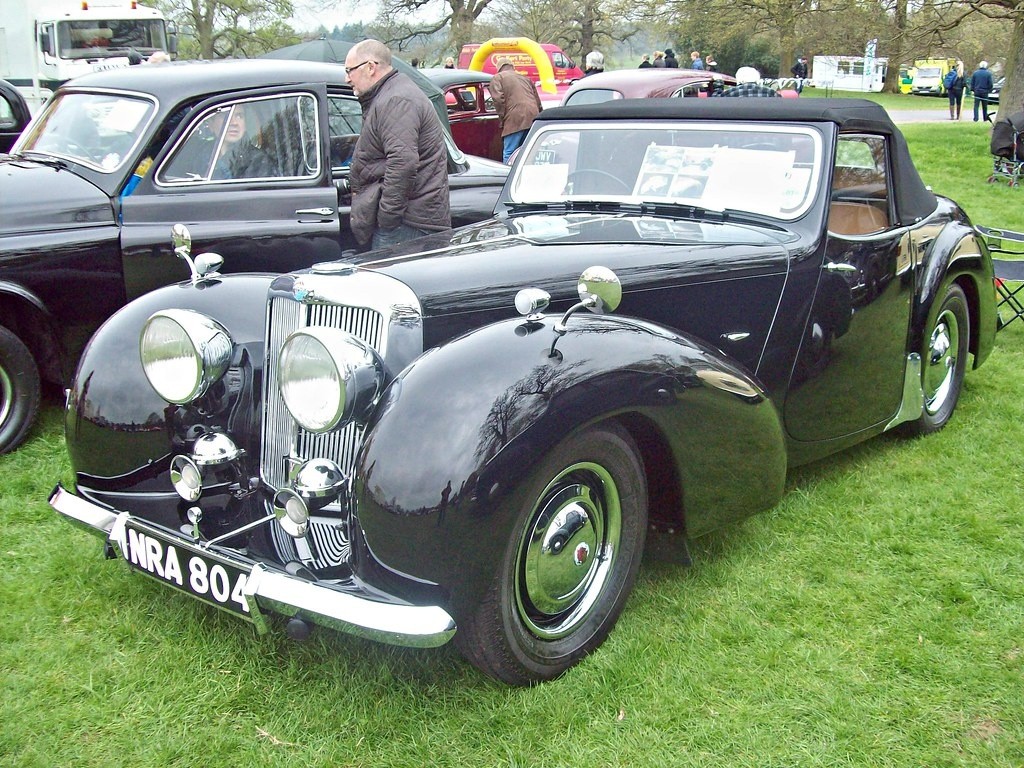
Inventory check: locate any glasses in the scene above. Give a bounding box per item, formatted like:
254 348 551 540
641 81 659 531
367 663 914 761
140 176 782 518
345 61 378 78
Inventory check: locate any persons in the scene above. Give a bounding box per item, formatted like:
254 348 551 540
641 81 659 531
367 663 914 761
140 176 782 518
344 38 451 250
790 56 808 94
489 58 543 165
193 104 272 178
971 61 993 121
585 52 606 77
638 53 653 68
948 64 964 120
690 51 704 70
653 51 666 68
711 66 783 97
664 49 678 68
705 55 722 73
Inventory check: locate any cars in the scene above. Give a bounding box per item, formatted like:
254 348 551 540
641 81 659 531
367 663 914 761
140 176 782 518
987 76 1006 105
409 69 563 165
506 67 739 173
47 85 999 689
0 56 569 457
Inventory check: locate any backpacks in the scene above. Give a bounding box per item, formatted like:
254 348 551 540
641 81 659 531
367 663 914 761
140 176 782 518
944 69 957 89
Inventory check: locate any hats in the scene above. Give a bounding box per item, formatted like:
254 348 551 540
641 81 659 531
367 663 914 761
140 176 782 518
497 59 512 72
665 49 672 54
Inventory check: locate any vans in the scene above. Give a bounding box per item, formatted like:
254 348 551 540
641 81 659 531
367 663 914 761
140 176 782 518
457 43 586 111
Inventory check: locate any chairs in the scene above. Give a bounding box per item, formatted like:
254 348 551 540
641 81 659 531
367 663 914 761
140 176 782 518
974 223 1024 331
827 202 887 235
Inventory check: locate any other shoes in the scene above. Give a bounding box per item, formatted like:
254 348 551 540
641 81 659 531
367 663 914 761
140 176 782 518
984 118 987 121
974 119 978 121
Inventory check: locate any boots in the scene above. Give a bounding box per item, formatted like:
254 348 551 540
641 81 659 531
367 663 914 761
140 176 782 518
950 105 954 120
957 106 960 119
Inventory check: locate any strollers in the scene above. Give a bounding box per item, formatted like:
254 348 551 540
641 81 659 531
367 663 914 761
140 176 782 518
985 110 1024 189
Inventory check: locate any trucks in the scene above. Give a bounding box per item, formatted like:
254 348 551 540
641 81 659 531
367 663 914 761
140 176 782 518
0 1 180 99
897 55 964 98
